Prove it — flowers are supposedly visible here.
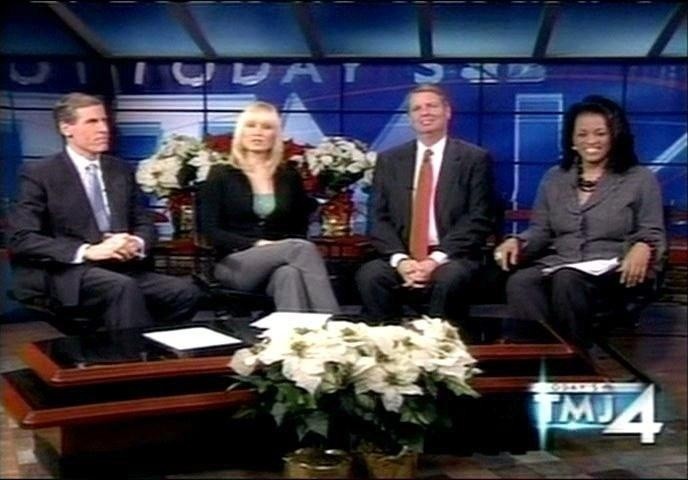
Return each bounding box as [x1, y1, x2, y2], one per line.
[289, 133, 380, 191]
[346, 318, 485, 453]
[130, 128, 238, 194]
[226, 317, 346, 440]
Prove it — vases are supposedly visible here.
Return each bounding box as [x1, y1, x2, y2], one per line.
[358, 436, 420, 479]
[281, 449, 355, 478]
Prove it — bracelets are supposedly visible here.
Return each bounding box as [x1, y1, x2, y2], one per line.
[631, 237, 657, 262]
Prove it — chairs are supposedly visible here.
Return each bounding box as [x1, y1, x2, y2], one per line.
[0, 180, 687, 346]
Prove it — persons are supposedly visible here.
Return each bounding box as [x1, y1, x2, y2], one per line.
[352, 83, 493, 323]
[494, 94, 667, 348]
[6, 93, 204, 330]
[197, 101, 340, 316]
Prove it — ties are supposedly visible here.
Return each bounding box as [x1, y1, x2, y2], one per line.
[409, 150, 433, 260]
[87, 165, 111, 233]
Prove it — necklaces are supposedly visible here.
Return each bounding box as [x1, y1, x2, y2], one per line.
[577, 166, 602, 192]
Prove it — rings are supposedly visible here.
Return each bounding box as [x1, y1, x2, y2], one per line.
[496, 252, 502, 260]
